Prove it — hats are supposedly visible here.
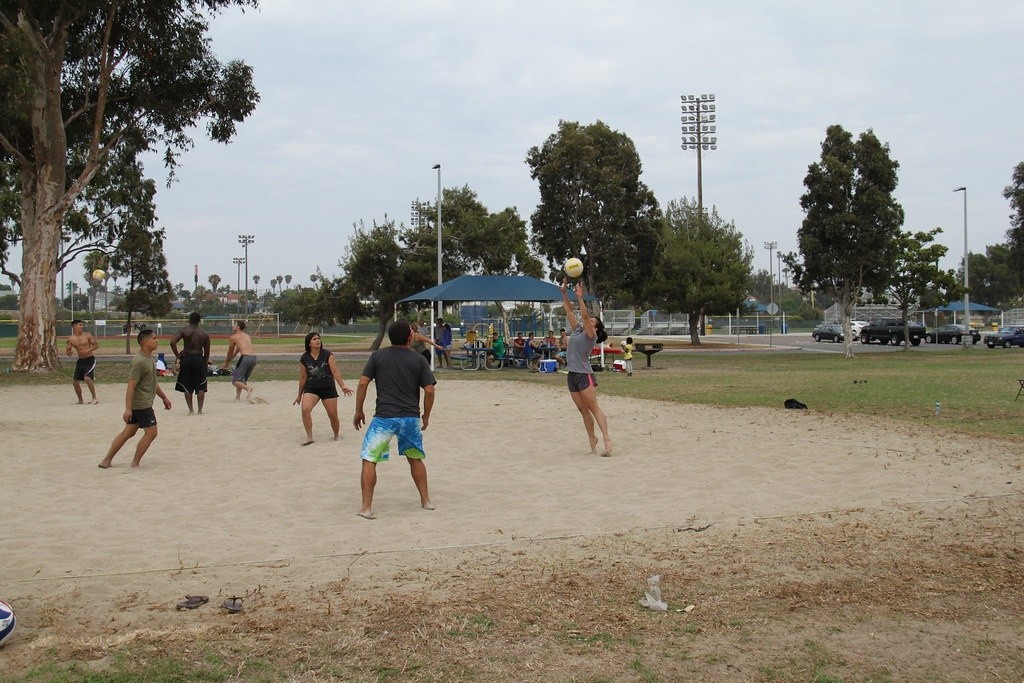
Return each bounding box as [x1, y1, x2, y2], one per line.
[436, 318, 443, 323]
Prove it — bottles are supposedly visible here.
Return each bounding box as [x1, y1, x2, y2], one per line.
[158, 353, 166, 368]
[474, 340, 477, 349]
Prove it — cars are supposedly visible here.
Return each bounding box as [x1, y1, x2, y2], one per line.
[983, 324, 1024, 349]
[924, 323, 982, 345]
[860, 317, 926, 347]
[840, 320, 871, 341]
[811, 323, 844, 342]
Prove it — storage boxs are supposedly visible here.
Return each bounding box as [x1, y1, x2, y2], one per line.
[540, 358, 557, 372]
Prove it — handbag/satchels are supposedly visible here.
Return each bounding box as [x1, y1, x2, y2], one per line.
[217, 369, 233, 377]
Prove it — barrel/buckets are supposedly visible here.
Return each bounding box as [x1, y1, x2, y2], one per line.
[467, 331, 476, 344]
[760, 324, 764, 333]
[706, 324, 712, 334]
[781, 324, 788, 334]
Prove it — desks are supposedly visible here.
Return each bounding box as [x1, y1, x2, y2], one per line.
[460, 346, 495, 371]
[590, 347, 622, 364]
[537, 347, 557, 361]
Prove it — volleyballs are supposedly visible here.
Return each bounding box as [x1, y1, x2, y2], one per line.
[564, 258, 583, 277]
[620, 341, 627, 346]
[93, 269, 106, 280]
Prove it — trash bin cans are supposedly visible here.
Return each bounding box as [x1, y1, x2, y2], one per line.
[781, 324, 788, 334]
[467, 330, 478, 344]
[760, 325, 764, 334]
[992, 322, 999, 331]
[706, 324, 713, 334]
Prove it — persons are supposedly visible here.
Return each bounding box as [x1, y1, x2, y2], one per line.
[353, 317, 436, 518]
[170, 312, 210, 415]
[98, 330, 171, 469]
[560, 278, 612, 457]
[66, 319, 99, 404]
[513, 333, 525, 355]
[543, 331, 556, 358]
[621, 337, 633, 376]
[123, 322, 141, 334]
[223, 320, 257, 400]
[552, 328, 569, 367]
[523, 332, 543, 368]
[471, 338, 485, 367]
[486, 332, 505, 368]
[410, 318, 452, 369]
[293, 332, 354, 446]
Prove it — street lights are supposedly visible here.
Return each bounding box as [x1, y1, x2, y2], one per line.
[432, 162, 444, 324]
[952, 186, 970, 334]
[680, 93, 718, 337]
[233, 257, 246, 319]
[763, 241, 778, 332]
[237, 235, 256, 324]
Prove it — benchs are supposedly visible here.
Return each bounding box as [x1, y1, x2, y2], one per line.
[451, 354, 535, 371]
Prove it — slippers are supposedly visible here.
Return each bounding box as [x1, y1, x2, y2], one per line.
[220, 595, 245, 614]
[98, 464, 107, 469]
[176, 594, 210, 612]
